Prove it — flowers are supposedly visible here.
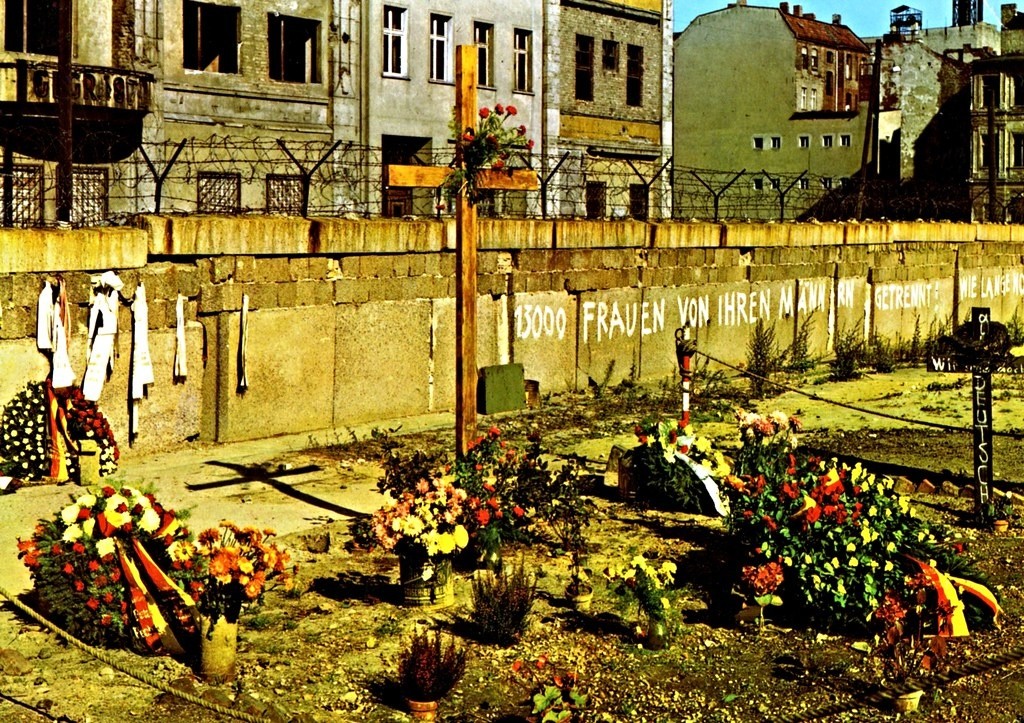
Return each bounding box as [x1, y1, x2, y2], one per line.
[565, 551, 593, 592]
[161, 521, 290, 639]
[355, 468, 468, 555]
[444, 103, 534, 194]
[375, 623, 470, 702]
[623, 560, 690, 613]
[876, 577, 955, 689]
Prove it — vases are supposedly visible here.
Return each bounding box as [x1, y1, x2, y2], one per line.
[410, 699, 437, 723]
[651, 618, 668, 648]
[892, 689, 923, 714]
[198, 617, 236, 682]
[400, 548, 454, 606]
[566, 586, 593, 613]
